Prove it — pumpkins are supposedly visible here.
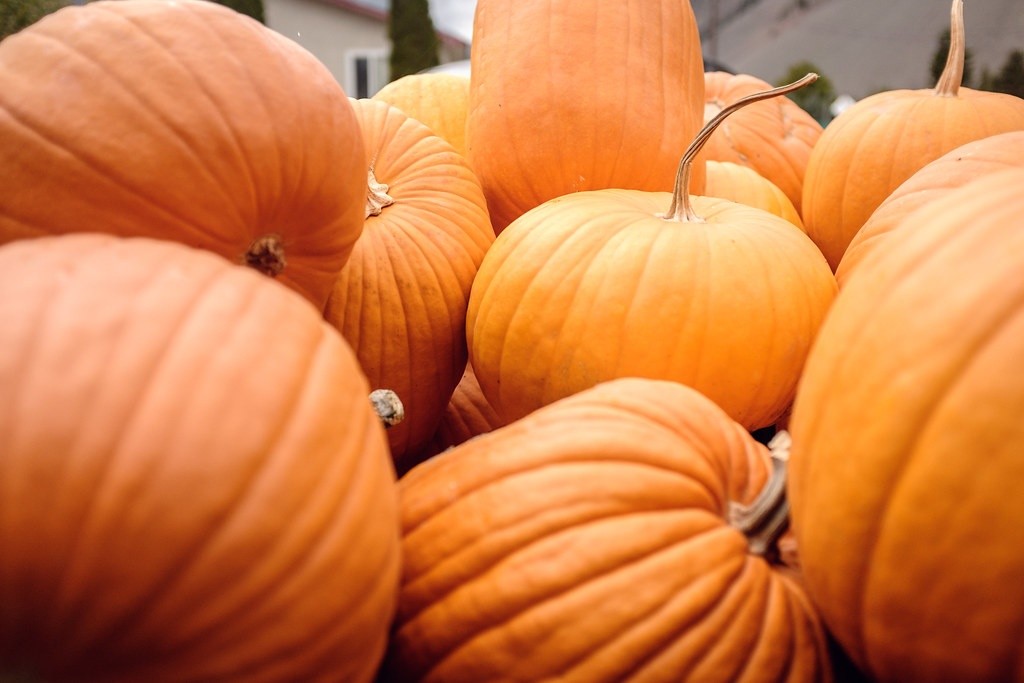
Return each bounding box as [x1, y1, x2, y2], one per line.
[0, 0, 1024, 683]
